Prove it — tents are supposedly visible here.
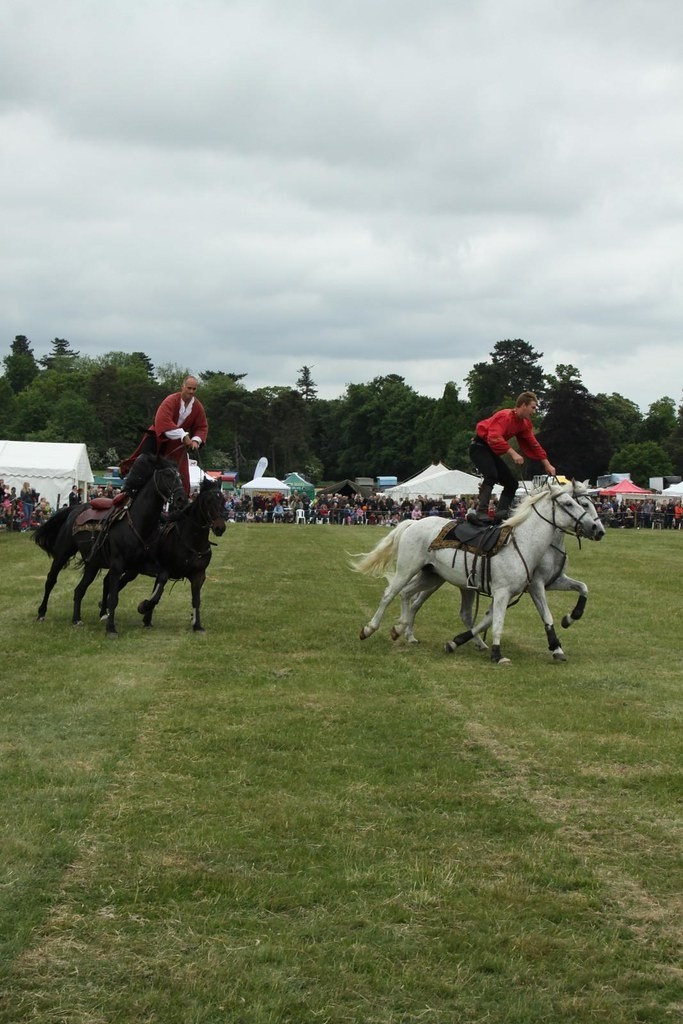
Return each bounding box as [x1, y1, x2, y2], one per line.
[0, 440, 95, 512]
[246, 462, 683, 508]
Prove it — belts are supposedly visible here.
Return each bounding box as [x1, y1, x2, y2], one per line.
[471, 438, 487, 446]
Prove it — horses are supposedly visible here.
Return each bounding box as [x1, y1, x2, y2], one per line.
[342, 478, 605, 668]
[29, 456, 228, 640]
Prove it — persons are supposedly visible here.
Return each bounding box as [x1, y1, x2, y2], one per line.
[153, 375, 209, 498]
[0, 478, 683, 532]
[468, 391, 558, 524]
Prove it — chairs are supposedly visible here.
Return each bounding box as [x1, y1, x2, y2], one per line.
[296, 509, 306, 524]
[650, 516, 662, 530]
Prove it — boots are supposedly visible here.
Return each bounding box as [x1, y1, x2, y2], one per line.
[476, 481, 493, 522]
[494, 495, 513, 524]
